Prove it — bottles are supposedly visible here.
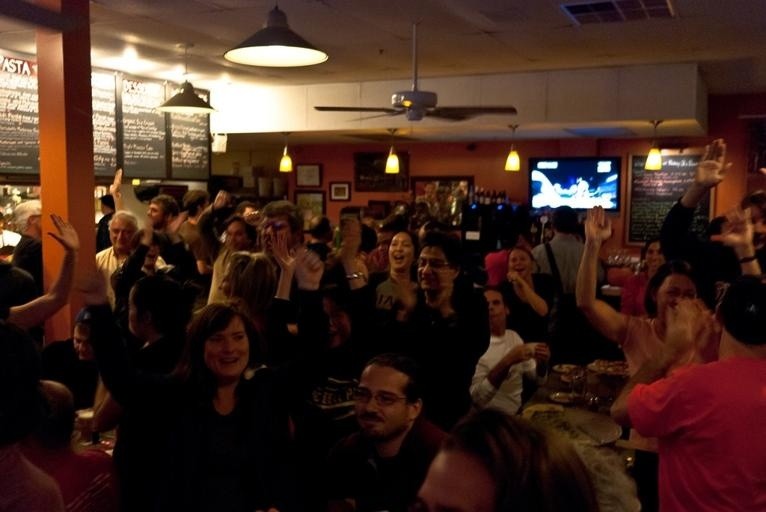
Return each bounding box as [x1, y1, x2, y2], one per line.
[467, 185, 506, 205]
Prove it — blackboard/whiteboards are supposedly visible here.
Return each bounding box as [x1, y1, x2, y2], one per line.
[625, 151, 716, 246]
[1, 49, 211, 184]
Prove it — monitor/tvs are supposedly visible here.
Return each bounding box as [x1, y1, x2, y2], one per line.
[528, 156, 622, 219]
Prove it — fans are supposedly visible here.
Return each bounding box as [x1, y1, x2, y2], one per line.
[311, 15, 517, 123]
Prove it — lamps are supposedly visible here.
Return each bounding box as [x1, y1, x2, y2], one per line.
[223, 0, 331, 67]
[154, 43, 218, 113]
[503, 125, 521, 172]
[277, 133, 293, 173]
[643, 120, 664, 171]
[383, 127, 400, 174]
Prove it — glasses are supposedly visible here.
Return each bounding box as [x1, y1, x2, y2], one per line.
[351, 386, 411, 408]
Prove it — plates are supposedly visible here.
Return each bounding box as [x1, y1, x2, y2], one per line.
[552, 363, 574, 372]
[587, 360, 627, 378]
[549, 391, 578, 403]
[520, 407, 623, 447]
[616, 438, 658, 453]
[258, 176, 287, 196]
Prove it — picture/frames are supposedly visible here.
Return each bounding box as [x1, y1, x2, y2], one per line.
[295, 163, 320, 186]
[412, 173, 474, 226]
[292, 190, 326, 221]
[328, 181, 351, 200]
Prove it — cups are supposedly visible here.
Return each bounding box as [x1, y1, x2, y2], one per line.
[570, 369, 587, 395]
[75, 407, 95, 447]
[527, 342, 540, 357]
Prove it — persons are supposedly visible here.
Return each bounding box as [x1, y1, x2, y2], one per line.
[657, 137, 766, 315]
[0, 166, 766, 512]
